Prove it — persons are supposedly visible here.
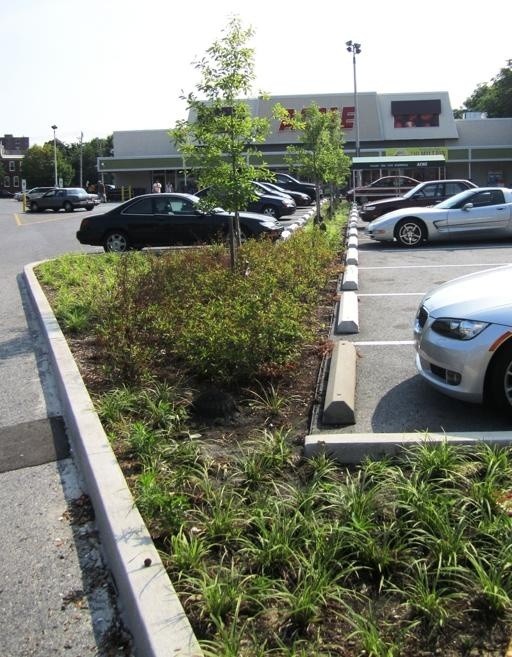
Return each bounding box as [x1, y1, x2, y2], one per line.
[180, 202, 193, 213]
[86, 180, 107, 203]
[152, 175, 173, 193]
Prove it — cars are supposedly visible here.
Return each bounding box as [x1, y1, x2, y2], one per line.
[0, 187, 14, 197]
[28, 186, 101, 212]
[191, 170, 325, 220]
[346, 174, 422, 204]
[360, 178, 483, 221]
[13, 188, 31, 201]
[413, 261, 512, 426]
[76, 191, 284, 252]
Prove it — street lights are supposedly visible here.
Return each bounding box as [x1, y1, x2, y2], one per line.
[51, 124, 60, 186]
[345, 38, 364, 188]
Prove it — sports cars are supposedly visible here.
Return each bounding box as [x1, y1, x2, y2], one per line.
[366, 186, 512, 247]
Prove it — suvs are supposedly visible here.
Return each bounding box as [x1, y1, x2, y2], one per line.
[19, 187, 60, 204]
